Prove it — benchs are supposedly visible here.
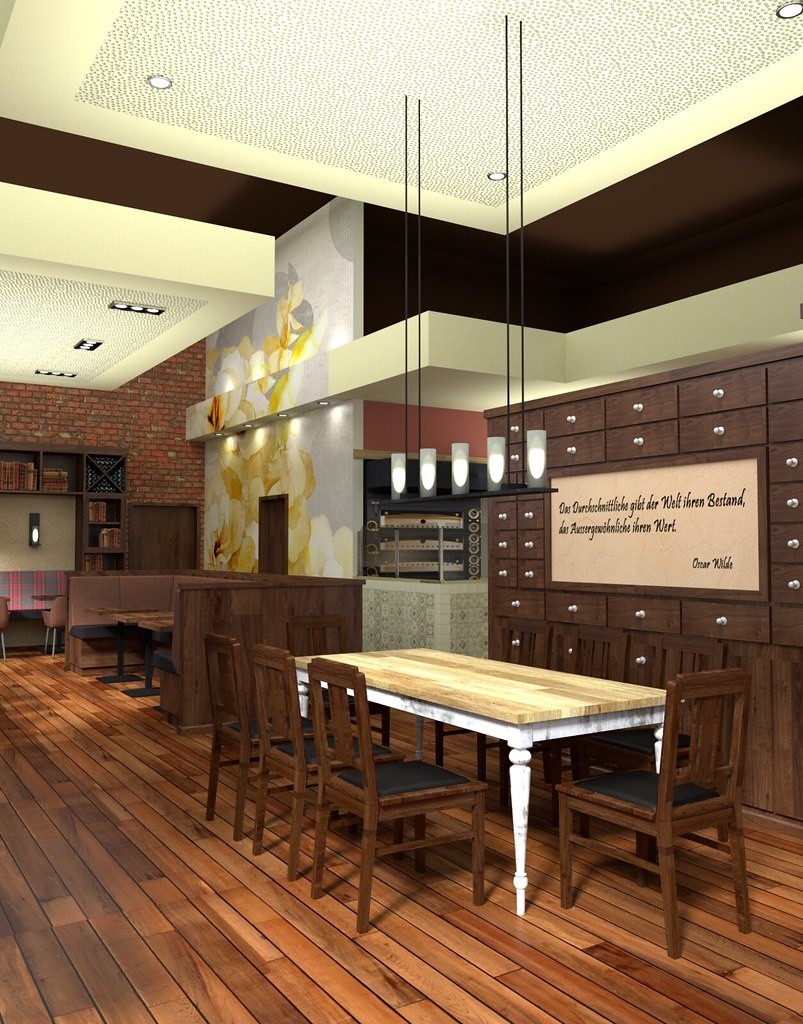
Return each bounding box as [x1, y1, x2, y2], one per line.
[64, 574, 366, 736]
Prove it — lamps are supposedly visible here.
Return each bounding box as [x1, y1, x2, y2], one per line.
[29, 513, 42, 547]
[377, 14, 559, 506]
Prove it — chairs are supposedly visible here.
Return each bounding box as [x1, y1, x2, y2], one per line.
[202, 614, 750, 960]
[42, 597, 68, 658]
[0, 598, 11, 662]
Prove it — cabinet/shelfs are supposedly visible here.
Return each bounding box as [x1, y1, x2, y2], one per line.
[0, 439, 129, 653]
[483, 343, 803, 822]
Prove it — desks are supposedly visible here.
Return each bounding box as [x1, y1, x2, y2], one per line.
[287, 646, 668, 920]
[111, 610, 174, 699]
[85, 607, 161, 685]
[138, 620, 174, 712]
[31, 595, 66, 654]
